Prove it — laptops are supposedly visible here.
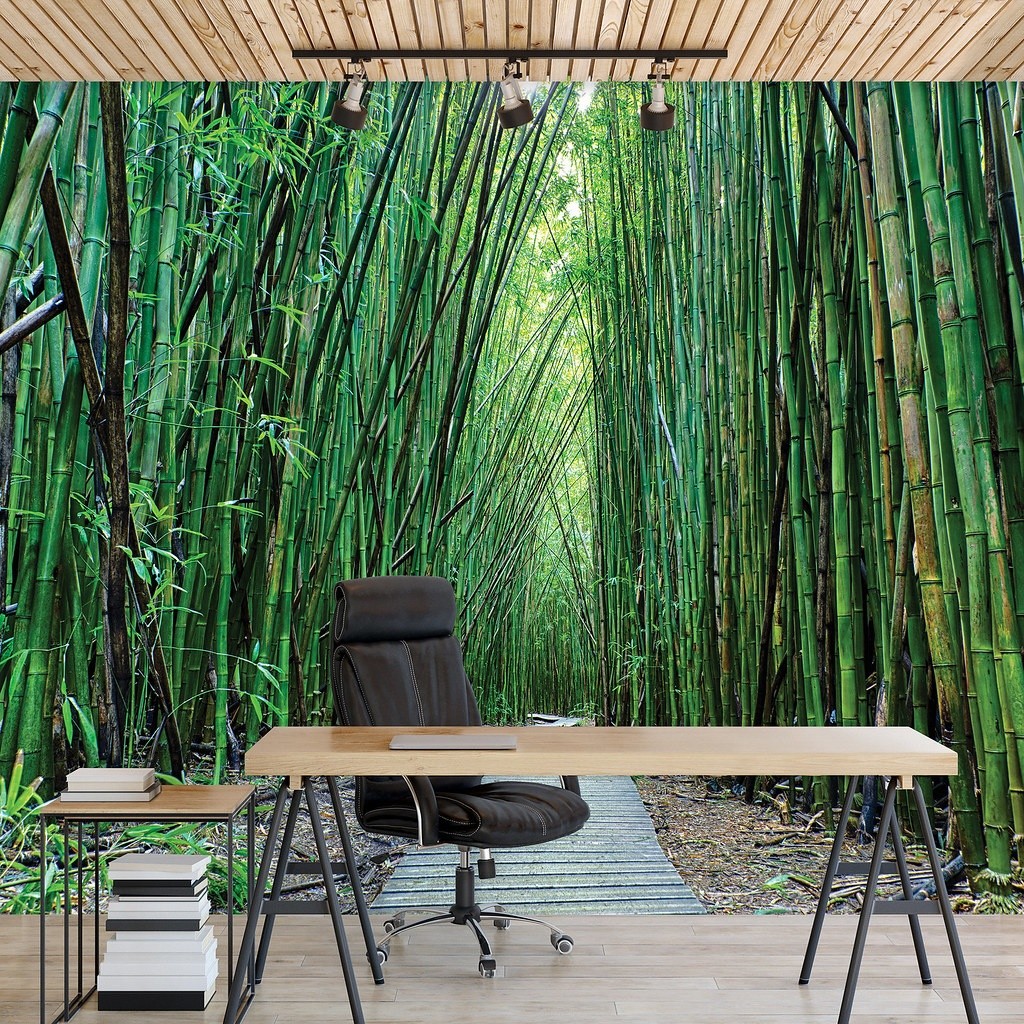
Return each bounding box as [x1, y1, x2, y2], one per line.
[389, 733, 516, 749]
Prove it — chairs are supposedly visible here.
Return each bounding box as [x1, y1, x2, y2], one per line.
[329, 575, 591, 978]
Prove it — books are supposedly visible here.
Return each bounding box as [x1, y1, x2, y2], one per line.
[97, 854, 219, 1011]
[60, 768, 162, 802]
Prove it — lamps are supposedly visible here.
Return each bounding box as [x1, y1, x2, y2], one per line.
[496, 57, 535, 130]
[332, 55, 367, 129]
[640, 57, 676, 131]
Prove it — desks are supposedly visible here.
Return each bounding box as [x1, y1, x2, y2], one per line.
[223, 724, 981, 1024]
[37, 785, 256, 1024]
